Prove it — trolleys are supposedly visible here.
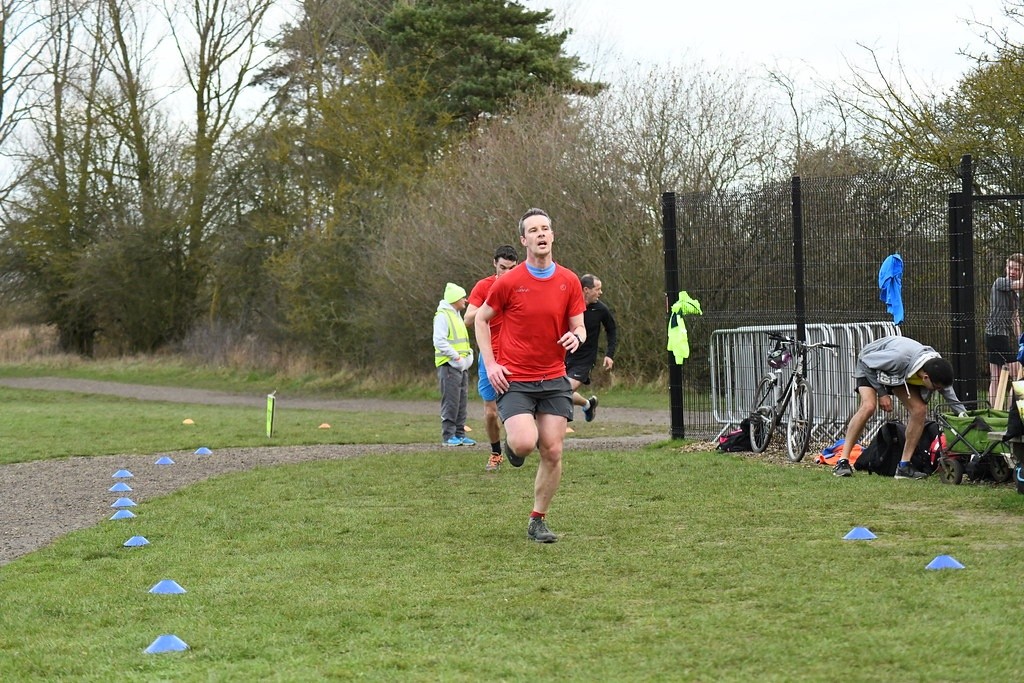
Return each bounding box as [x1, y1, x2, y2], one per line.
[935, 400, 1013, 483]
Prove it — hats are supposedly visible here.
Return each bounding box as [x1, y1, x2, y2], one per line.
[443, 283, 467, 304]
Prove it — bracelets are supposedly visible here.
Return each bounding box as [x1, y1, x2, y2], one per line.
[574, 334, 583, 346]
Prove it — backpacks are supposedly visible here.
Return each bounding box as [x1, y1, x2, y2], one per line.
[719, 417, 763, 453]
[852, 420, 947, 477]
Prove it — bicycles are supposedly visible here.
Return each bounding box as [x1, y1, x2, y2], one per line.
[749, 329, 841, 462]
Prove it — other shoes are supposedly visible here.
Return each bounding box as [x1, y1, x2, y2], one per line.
[565, 426, 577, 433]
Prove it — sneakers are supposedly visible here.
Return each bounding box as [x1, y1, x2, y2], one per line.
[582, 395, 598, 422]
[528, 515, 558, 542]
[442, 436, 464, 446]
[485, 451, 504, 472]
[457, 437, 476, 446]
[505, 439, 525, 467]
[893, 461, 928, 480]
[833, 457, 852, 477]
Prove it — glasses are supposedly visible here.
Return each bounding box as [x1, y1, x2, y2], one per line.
[928, 375, 944, 391]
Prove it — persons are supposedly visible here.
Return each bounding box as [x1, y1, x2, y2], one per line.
[432, 282, 477, 446]
[563, 273, 617, 422]
[831, 332, 968, 480]
[463, 245, 518, 472]
[984, 253, 1024, 411]
[474, 207, 588, 542]
